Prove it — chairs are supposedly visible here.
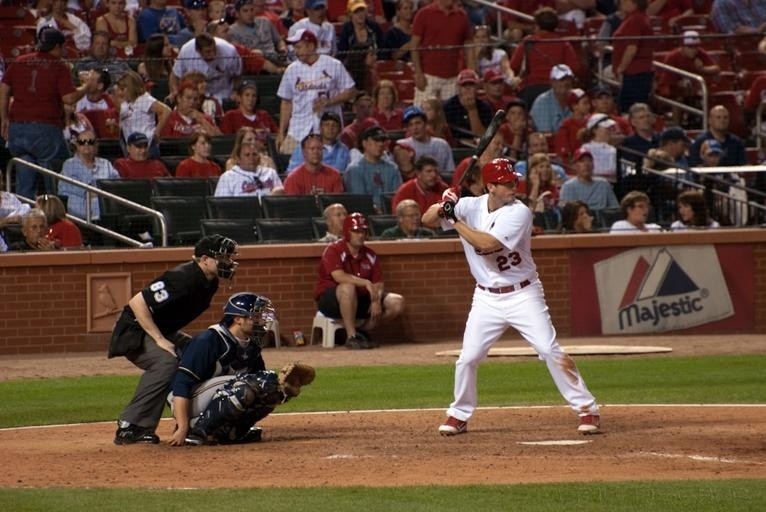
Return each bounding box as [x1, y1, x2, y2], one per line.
[1, 0, 766, 252]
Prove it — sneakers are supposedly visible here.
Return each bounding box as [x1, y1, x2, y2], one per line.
[113, 418, 160, 445]
[221, 426, 265, 445]
[346, 329, 380, 351]
[577, 413, 601, 434]
[437, 416, 468, 436]
[172, 425, 220, 446]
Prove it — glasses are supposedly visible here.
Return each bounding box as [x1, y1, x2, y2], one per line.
[74, 138, 99, 146]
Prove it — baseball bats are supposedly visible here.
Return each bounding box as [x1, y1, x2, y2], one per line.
[437, 109, 506, 220]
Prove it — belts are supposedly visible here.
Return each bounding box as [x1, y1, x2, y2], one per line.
[476, 279, 531, 296]
[7, 118, 59, 126]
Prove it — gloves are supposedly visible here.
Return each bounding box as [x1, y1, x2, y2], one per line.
[441, 186, 462, 226]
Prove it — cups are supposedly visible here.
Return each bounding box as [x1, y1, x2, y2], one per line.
[311, 99, 322, 114]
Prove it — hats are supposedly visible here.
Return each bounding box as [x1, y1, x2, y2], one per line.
[565, 84, 615, 108]
[125, 130, 149, 145]
[33, 26, 58, 53]
[457, 70, 481, 87]
[303, 0, 328, 11]
[569, 148, 594, 165]
[483, 67, 505, 83]
[175, 0, 263, 96]
[586, 112, 617, 133]
[355, 103, 428, 159]
[662, 126, 696, 145]
[321, 112, 342, 127]
[348, 0, 368, 13]
[681, 30, 702, 47]
[550, 63, 576, 81]
[699, 138, 727, 158]
[284, 28, 318, 46]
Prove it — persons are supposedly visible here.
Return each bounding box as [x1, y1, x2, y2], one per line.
[420, 156, 605, 435]
[316, 211, 403, 353]
[106, 234, 241, 446]
[165, 290, 318, 450]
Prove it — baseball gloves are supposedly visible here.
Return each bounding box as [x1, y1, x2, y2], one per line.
[275, 365, 316, 401]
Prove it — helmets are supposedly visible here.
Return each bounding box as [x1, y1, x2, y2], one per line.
[223, 292, 275, 345]
[481, 157, 523, 188]
[192, 233, 241, 280]
[342, 211, 371, 242]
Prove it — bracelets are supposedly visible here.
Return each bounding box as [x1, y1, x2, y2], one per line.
[448, 215, 461, 225]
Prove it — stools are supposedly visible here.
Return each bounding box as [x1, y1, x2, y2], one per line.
[308, 309, 366, 348]
[264, 319, 282, 348]
